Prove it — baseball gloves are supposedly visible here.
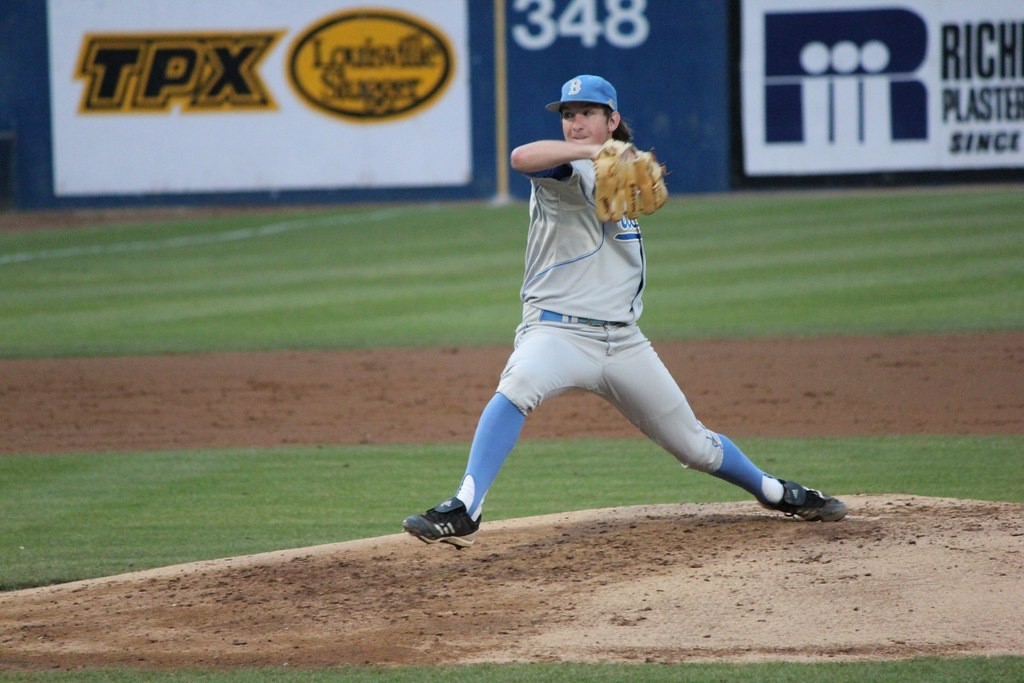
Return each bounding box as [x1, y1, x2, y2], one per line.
[592, 139, 669, 223]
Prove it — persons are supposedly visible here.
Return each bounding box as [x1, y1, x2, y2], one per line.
[403, 75, 848, 551]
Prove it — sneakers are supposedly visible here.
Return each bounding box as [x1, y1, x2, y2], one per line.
[402, 498, 482, 550]
[758, 477, 848, 521]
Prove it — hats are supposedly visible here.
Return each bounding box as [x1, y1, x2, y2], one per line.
[544, 74, 617, 113]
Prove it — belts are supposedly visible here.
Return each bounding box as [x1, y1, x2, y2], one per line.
[540, 310, 614, 327]
